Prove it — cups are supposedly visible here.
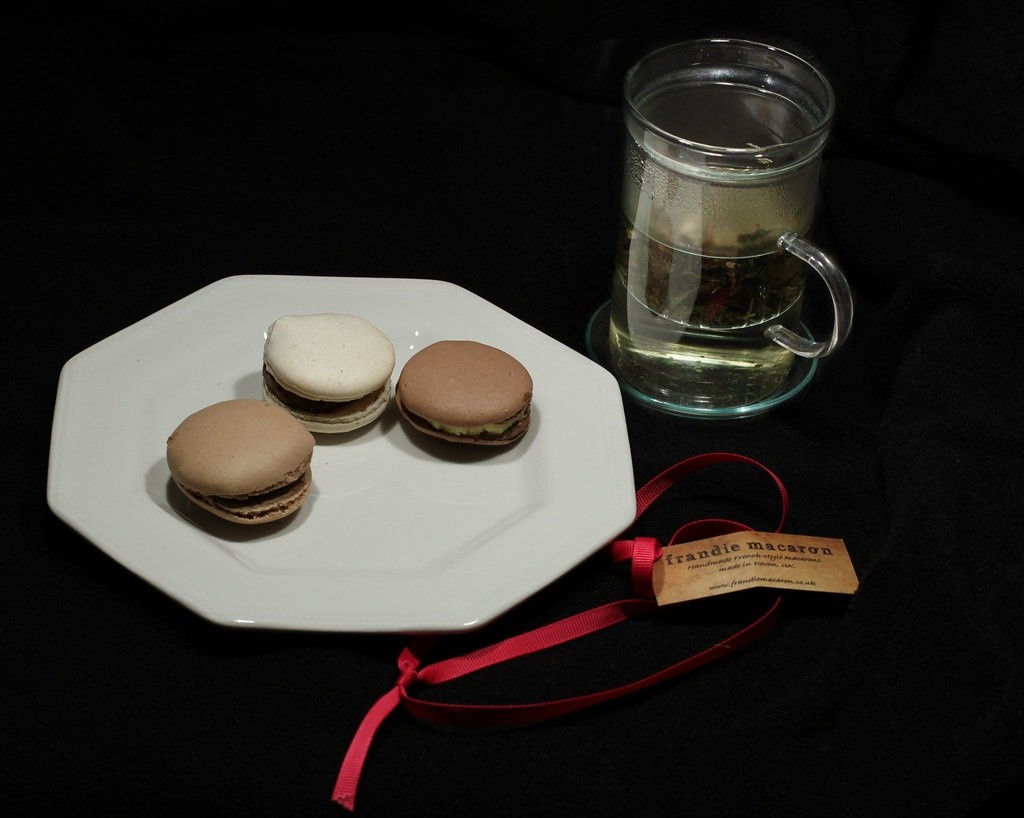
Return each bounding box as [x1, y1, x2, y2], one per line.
[605, 36, 854, 408]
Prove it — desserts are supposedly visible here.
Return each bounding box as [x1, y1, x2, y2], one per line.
[263, 314, 393, 434]
[166, 400, 316, 525]
[399, 340, 532, 458]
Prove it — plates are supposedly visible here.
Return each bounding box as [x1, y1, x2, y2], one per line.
[46, 275, 639, 632]
